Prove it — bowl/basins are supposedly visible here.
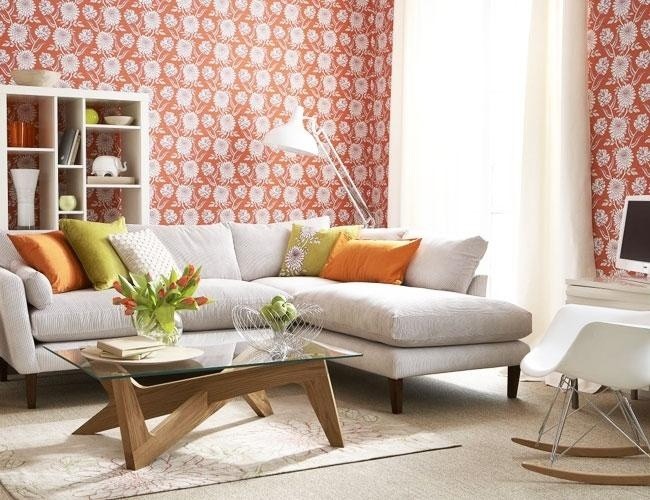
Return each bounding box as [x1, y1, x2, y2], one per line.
[12, 68, 61, 87]
[104, 116, 135, 126]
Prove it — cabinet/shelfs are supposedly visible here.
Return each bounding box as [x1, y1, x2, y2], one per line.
[0, 84, 150, 231]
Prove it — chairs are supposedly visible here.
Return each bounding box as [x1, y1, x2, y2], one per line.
[510, 301, 650, 487]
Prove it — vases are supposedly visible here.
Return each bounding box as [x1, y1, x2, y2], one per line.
[134, 310, 182, 345]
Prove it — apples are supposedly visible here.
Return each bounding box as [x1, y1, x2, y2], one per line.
[259, 297, 296, 333]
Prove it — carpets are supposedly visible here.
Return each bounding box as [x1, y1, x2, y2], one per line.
[1, 393, 463, 500]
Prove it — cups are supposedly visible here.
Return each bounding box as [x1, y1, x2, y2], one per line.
[59, 195, 78, 211]
[8, 119, 34, 147]
[7, 168, 41, 229]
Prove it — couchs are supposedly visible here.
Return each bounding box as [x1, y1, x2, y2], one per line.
[1, 225, 533, 414]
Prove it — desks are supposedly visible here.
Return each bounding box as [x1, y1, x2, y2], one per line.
[566, 279, 650, 409]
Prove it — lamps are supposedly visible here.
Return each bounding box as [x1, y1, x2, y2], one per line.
[263, 106, 376, 233]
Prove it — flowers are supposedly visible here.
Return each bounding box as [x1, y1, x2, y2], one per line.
[111, 264, 210, 321]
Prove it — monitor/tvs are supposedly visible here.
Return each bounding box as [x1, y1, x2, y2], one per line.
[615, 195, 650, 283]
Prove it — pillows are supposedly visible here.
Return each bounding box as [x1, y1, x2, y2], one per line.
[10, 217, 183, 294]
[278, 223, 423, 286]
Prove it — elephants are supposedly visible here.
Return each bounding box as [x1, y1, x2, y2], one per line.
[90, 155, 127, 177]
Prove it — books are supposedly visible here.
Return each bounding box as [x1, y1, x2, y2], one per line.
[97, 335, 165, 356]
[102, 350, 152, 361]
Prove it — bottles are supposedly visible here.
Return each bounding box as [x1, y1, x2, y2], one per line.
[86, 108, 98, 124]
